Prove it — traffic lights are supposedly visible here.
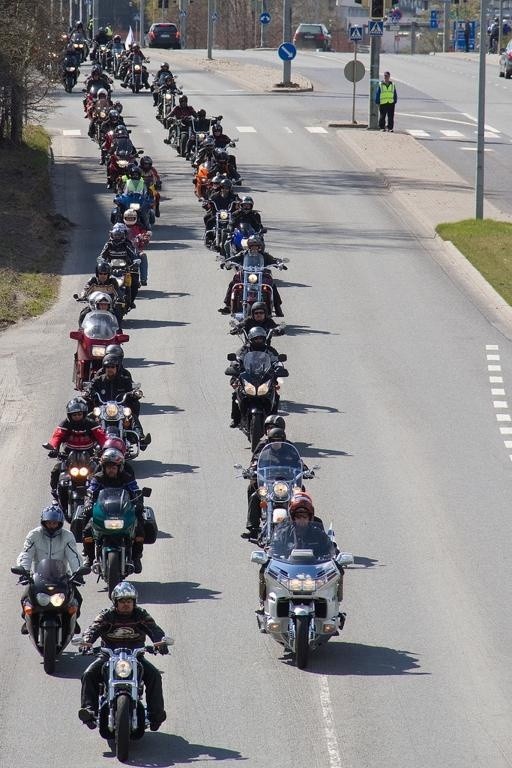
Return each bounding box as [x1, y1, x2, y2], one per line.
[158, 0, 162, 8]
[164, 0, 168, 8]
[385, 0, 400, 9]
[453, 0, 461, 6]
[462, 0, 468, 4]
[354, 0, 368, 7]
[370, 0, 384, 19]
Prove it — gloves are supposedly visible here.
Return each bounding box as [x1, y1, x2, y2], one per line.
[155, 644, 169, 655]
[48, 449, 58, 458]
[84, 499, 92, 514]
[303, 469, 314, 479]
[79, 645, 89, 656]
[135, 503, 143, 516]
[72, 572, 83, 587]
[19, 570, 30, 585]
[244, 466, 257, 480]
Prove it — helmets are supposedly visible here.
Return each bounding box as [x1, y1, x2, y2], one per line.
[91, 64, 129, 138]
[111, 582, 138, 606]
[41, 505, 64, 530]
[211, 177, 254, 215]
[287, 492, 313, 528]
[66, 397, 88, 422]
[101, 437, 127, 473]
[97, 263, 111, 280]
[248, 235, 264, 251]
[126, 156, 152, 179]
[264, 415, 286, 451]
[247, 326, 267, 347]
[89, 291, 113, 312]
[251, 302, 268, 322]
[160, 62, 187, 106]
[66, 21, 83, 54]
[102, 344, 124, 374]
[109, 208, 137, 243]
[196, 109, 222, 150]
[98, 27, 139, 53]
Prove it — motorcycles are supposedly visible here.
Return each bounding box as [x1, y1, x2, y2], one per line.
[225, 351, 287, 452]
[75, 484, 157, 598]
[70, 311, 132, 389]
[249, 522, 353, 668]
[233, 442, 321, 548]
[87, 390, 145, 464]
[43, 20, 150, 92]
[9, 558, 93, 675]
[42, 441, 109, 522]
[68, 634, 175, 761]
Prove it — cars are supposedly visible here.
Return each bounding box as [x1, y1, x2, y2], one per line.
[498, 39, 512, 78]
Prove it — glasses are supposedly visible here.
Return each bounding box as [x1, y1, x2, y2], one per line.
[119, 598, 132, 603]
[72, 413, 81, 416]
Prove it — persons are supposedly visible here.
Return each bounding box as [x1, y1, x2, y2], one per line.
[201, 174, 287, 357]
[229, 326, 343, 614]
[77, 155, 162, 332]
[77, 581, 168, 731]
[16, 345, 145, 636]
[28, 14, 149, 88]
[375, 71, 397, 132]
[456, 14, 512, 55]
[82, 61, 241, 187]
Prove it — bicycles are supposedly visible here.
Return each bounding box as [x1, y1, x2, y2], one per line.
[490, 37, 498, 54]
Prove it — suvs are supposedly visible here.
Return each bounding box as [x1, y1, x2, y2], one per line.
[146, 23, 181, 49]
[293, 23, 332, 51]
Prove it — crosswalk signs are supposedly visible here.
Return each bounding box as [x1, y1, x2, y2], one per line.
[350, 27, 363, 40]
[368, 20, 383, 36]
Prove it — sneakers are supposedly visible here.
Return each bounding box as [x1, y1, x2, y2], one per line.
[205, 233, 229, 258]
[79, 705, 97, 729]
[21, 622, 29, 634]
[150, 711, 165, 731]
[132, 553, 142, 573]
[74, 621, 81, 634]
[52, 497, 58, 505]
[338, 612, 346, 625]
[99, 153, 121, 194]
[230, 418, 240, 427]
[82, 549, 95, 567]
[140, 433, 150, 451]
[274, 305, 283, 316]
[217, 305, 231, 313]
[153, 101, 171, 129]
[163, 137, 195, 162]
[241, 527, 257, 539]
[255, 608, 265, 615]
